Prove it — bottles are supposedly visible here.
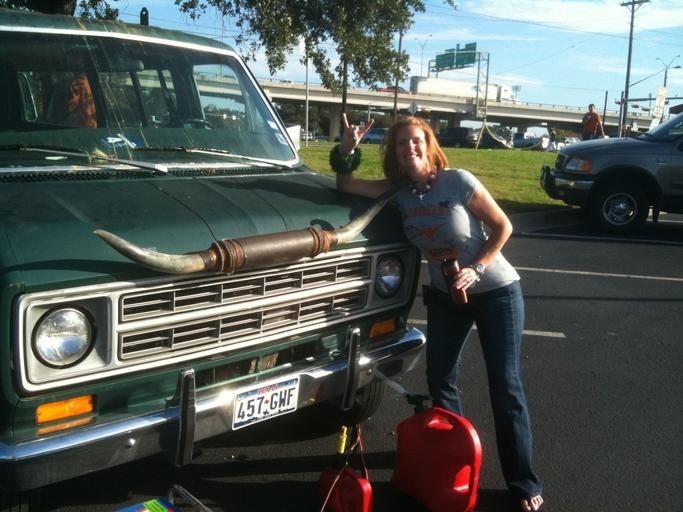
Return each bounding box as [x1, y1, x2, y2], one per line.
[441, 257, 470, 307]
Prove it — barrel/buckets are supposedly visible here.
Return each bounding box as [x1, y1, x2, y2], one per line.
[319, 463, 374, 512]
[391, 393, 482, 512]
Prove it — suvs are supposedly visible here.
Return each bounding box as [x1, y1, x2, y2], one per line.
[538, 112, 683, 237]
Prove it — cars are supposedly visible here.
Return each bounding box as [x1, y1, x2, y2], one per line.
[379, 83, 410, 95]
[280, 123, 578, 156]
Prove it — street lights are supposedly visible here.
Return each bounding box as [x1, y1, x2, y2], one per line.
[654, 55, 679, 89]
[411, 33, 432, 76]
[616, 66, 682, 137]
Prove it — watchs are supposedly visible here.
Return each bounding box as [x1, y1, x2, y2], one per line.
[463, 263, 486, 276]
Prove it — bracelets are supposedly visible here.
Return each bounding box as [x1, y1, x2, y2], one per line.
[329, 145, 362, 174]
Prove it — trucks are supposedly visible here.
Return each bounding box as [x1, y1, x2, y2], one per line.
[410, 76, 520, 104]
[0, 2, 425, 499]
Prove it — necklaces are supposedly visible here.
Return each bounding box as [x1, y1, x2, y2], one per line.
[402, 165, 438, 203]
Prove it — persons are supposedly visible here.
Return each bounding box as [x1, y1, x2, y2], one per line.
[335, 108, 545, 512]
[580, 103, 604, 141]
